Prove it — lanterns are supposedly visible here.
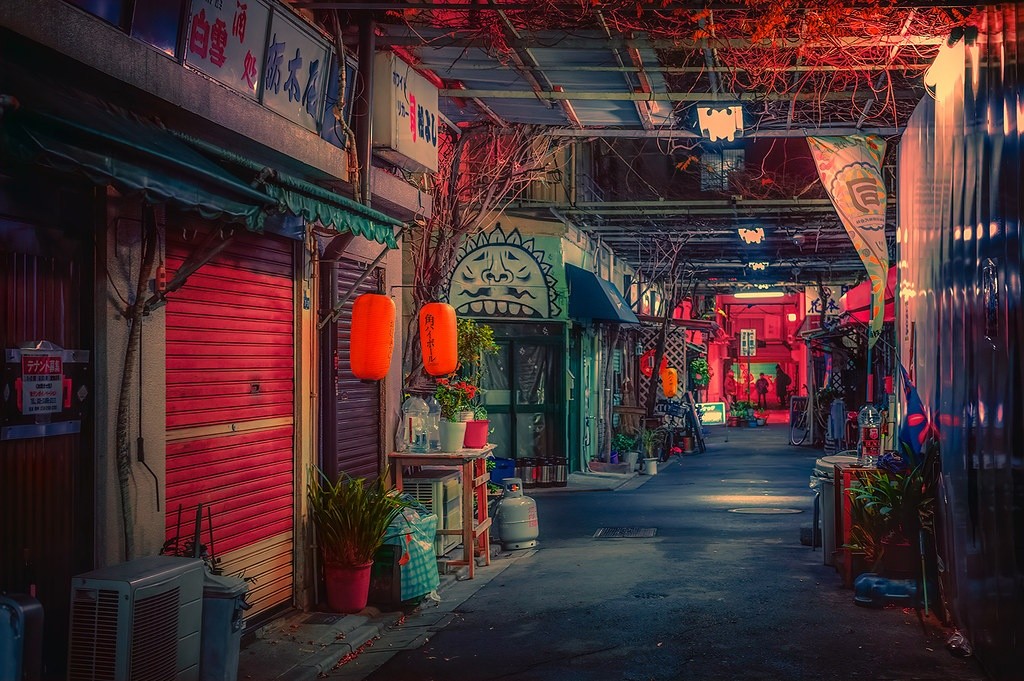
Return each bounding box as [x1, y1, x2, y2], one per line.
[419, 300, 458, 384]
[350, 290, 396, 385]
[662, 366, 677, 399]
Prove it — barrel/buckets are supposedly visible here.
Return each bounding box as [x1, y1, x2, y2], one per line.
[463, 420, 491, 448]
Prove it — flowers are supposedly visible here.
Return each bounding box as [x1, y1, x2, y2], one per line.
[432, 377, 478, 422]
[697, 278, 728, 323]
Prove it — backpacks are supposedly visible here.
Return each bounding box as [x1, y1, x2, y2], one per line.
[784, 373, 792, 386]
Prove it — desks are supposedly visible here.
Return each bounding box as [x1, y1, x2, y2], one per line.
[834, 462, 915, 590]
[380, 501, 439, 609]
[388, 442, 497, 578]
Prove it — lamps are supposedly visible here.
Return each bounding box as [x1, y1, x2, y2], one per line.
[738, 228, 766, 244]
[696, 103, 744, 143]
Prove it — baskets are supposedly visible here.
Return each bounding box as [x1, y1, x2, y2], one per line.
[795, 396, 808, 412]
[666, 403, 686, 419]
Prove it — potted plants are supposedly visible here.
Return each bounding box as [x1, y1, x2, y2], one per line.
[611, 434, 639, 473]
[434, 317, 501, 449]
[840, 441, 942, 577]
[303, 462, 425, 612]
[635, 426, 665, 475]
[728, 400, 766, 428]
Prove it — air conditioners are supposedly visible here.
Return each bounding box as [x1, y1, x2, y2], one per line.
[67, 553, 204, 681]
[402, 468, 464, 559]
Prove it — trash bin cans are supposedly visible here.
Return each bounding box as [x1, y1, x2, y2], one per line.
[202, 572, 254, 681]
[817, 456, 859, 568]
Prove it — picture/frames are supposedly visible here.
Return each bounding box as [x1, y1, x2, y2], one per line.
[697, 402, 726, 426]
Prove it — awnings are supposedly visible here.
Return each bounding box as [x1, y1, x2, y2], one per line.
[564, 261, 640, 324]
[0, 88, 409, 318]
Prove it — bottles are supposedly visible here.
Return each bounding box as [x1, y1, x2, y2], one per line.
[402, 392, 443, 455]
[856, 401, 882, 470]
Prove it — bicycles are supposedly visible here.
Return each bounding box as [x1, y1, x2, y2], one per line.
[777, 386, 798, 408]
[652, 396, 686, 462]
[791, 384, 827, 445]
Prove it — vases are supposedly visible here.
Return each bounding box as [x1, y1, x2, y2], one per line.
[438, 420, 466, 452]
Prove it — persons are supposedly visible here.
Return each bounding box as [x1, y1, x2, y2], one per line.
[755, 374, 769, 410]
[723, 370, 738, 402]
[775, 364, 786, 407]
